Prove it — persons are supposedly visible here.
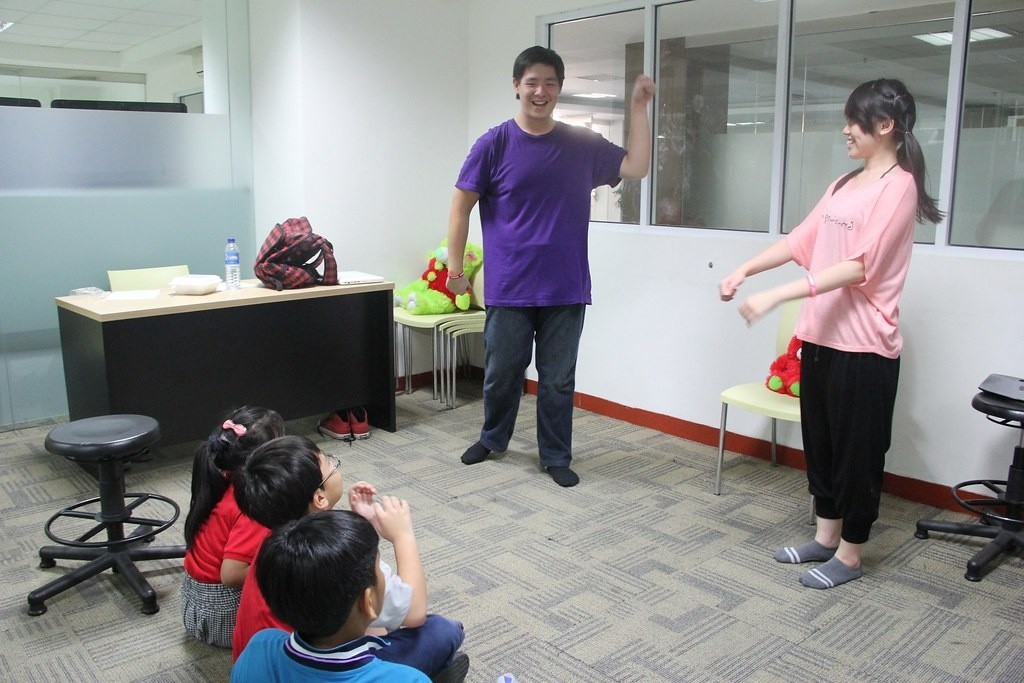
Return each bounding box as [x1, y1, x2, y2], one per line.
[444, 46, 657, 488]
[718, 78, 947, 591]
[182, 406, 470, 683]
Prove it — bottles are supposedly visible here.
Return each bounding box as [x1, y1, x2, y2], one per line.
[225, 238, 240, 291]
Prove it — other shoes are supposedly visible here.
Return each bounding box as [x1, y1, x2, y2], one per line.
[431, 651, 469, 683]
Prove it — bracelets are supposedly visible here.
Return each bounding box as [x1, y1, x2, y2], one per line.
[448, 270, 464, 280]
[806, 274, 817, 298]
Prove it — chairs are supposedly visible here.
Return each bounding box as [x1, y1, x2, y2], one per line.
[714, 297, 816, 525]
[107, 265, 190, 291]
[392, 262, 525, 410]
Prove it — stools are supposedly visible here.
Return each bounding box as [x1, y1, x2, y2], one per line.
[915, 392, 1024, 582]
[26, 415, 186, 618]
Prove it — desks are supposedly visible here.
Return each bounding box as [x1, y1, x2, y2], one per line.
[53, 271, 397, 483]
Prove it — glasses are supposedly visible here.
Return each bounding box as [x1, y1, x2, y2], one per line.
[319, 449, 341, 487]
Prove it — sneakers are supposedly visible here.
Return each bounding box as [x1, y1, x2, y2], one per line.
[316, 406, 352, 439]
[346, 407, 370, 439]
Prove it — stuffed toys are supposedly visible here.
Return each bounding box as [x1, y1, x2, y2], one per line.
[766, 336, 803, 398]
[392, 235, 484, 316]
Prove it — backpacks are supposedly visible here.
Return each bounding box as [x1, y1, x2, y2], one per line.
[254, 217, 337, 290]
